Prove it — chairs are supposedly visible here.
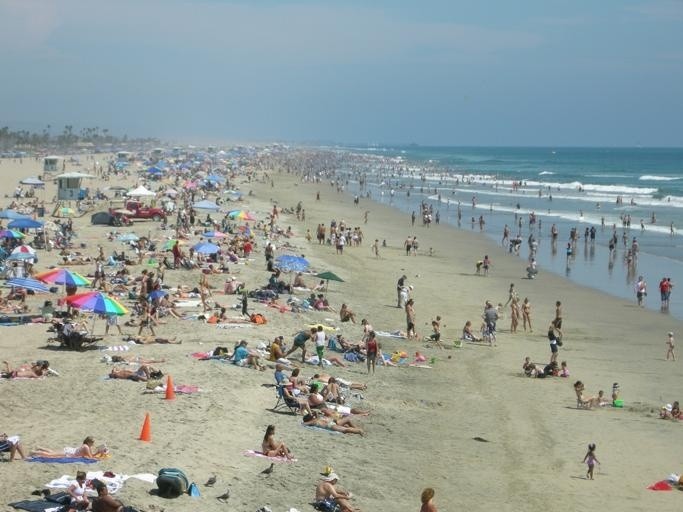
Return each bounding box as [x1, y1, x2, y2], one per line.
[47, 320, 102, 351]
[0, 301, 40, 325]
[271, 388, 301, 415]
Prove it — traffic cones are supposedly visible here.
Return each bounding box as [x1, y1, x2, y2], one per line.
[139, 413, 153, 442]
[165, 375, 177, 400]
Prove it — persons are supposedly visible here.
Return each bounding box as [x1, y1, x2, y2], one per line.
[1, 144, 683, 512]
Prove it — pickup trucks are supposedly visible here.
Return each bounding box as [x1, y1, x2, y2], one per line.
[109, 200, 165, 222]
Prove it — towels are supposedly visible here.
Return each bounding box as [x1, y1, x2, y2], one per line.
[27, 450, 111, 465]
[160, 279, 294, 331]
[297, 310, 410, 369]
[142, 382, 203, 395]
[243, 449, 298, 463]
[297, 420, 341, 438]
[8, 498, 68, 512]
[43, 471, 121, 496]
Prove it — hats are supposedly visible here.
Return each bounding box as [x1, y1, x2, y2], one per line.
[421, 488, 434, 502]
[279, 379, 292, 385]
[326, 473, 338, 481]
